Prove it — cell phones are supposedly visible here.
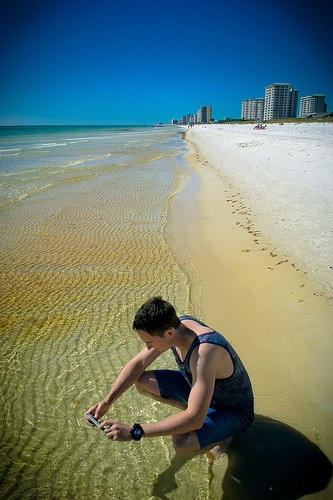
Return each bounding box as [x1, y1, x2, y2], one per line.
[87, 412, 106, 430]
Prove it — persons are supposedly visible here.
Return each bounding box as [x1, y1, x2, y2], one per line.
[84, 297, 256, 461]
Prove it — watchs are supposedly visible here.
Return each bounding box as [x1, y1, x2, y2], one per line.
[129, 422, 144, 442]
